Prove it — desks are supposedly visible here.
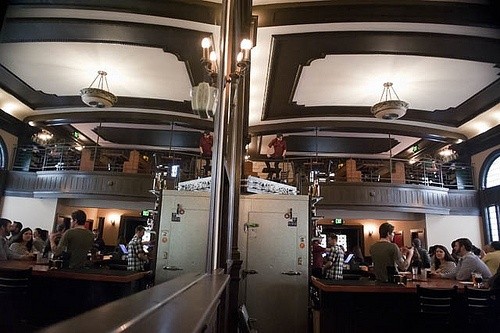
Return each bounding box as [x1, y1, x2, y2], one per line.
[0, 259, 152, 287]
[310, 279, 480, 333]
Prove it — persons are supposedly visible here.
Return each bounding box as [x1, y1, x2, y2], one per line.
[48, 210, 96, 272]
[370, 222, 414, 283]
[339, 245, 365, 270]
[114, 224, 148, 271]
[398, 240, 500, 282]
[0, 210, 104, 274]
[310, 237, 333, 298]
[324, 234, 344, 279]
[199, 132, 213, 174]
[265, 133, 287, 180]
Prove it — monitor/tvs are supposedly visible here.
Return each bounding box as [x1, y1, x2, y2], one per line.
[120, 244, 128, 254]
[344, 254, 354, 263]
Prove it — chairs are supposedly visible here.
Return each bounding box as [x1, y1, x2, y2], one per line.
[463, 285, 493, 333]
[243, 160, 257, 179]
[416, 285, 457, 333]
[316, 159, 332, 183]
[0, 267, 32, 328]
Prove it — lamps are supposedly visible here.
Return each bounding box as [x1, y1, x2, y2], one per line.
[200, 35, 218, 81]
[229, 38, 254, 83]
[370, 82, 409, 120]
[80, 71, 119, 109]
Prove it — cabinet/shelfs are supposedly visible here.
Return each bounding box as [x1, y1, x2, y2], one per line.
[123, 151, 155, 174]
[79, 148, 107, 171]
[336, 159, 362, 182]
[379, 161, 405, 184]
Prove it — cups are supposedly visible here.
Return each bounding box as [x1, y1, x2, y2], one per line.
[471, 273, 476, 286]
[475, 272, 482, 285]
[421, 268, 427, 279]
[412, 266, 418, 275]
